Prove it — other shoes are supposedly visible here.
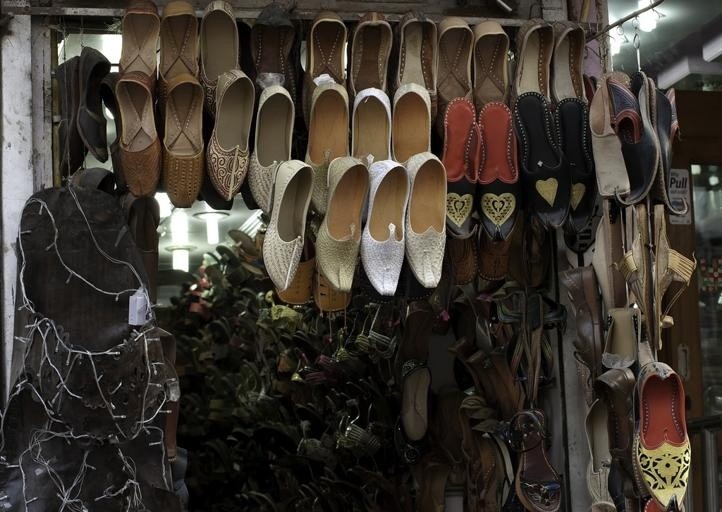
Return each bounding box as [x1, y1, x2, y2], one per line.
[314, 155, 370, 294]
[251, 3, 299, 112]
[473, 20, 511, 120]
[301, 7, 346, 127]
[351, 88, 392, 168]
[549, 20, 589, 109]
[164, 2, 200, 82]
[205, 69, 256, 204]
[249, 85, 296, 216]
[440, 18, 474, 117]
[403, 152, 448, 289]
[171, 231, 634, 510]
[262, 160, 315, 293]
[119, 5, 161, 101]
[200, 1, 243, 120]
[398, 11, 439, 119]
[350, 11, 396, 97]
[637, 360, 691, 509]
[360, 159, 411, 297]
[115, 72, 162, 200]
[392, 82, 433, 164]
[305, 82, 351, 216]
[161, 74, 205, 208]
[588, 69, 699, 351]
[511, 18, 555, 109]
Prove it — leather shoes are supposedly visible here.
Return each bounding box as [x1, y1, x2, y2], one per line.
[55, 56, 88, 177]
[71, 164, 117, 198]
[75, 45, 115, 164]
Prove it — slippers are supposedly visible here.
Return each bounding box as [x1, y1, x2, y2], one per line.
[479, 102, 519, 246]
[555, 97, 598, 231]
[440, 97, 478, 239]
[514, 91, 573, 232]
